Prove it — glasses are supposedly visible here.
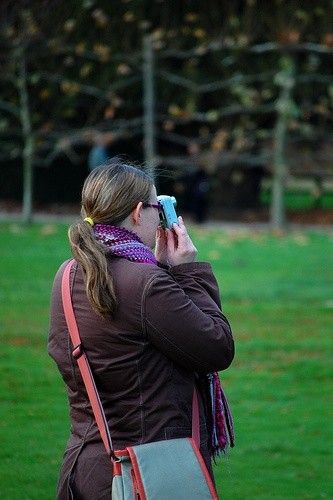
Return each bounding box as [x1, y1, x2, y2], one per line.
[143, 202, 163, 213]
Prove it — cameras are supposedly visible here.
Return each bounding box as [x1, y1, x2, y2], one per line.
[157, 195, 179, 231]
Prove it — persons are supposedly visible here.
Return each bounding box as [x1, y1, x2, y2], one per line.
[48, 163, 236, 499]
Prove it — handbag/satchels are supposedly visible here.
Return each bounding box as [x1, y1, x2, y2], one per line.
[111, 437, 218, 500]
